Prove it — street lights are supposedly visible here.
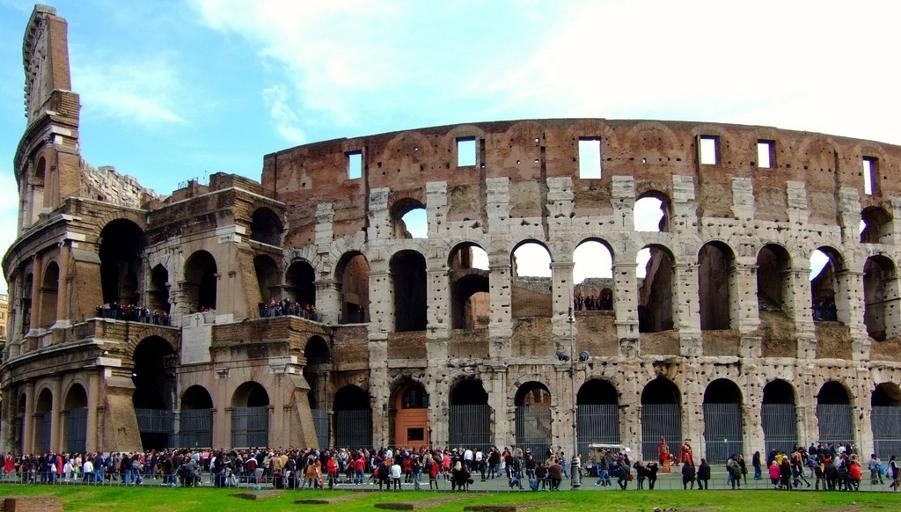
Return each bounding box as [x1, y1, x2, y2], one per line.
[326, 410, 335, 450]
[555, 304, 590, 489]
[425, 419, 432, 450]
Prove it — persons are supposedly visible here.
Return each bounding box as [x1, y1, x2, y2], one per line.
[0, 440, 900, 491]
[263, 296, 319, 323]
[94, 302, 169, 327]
